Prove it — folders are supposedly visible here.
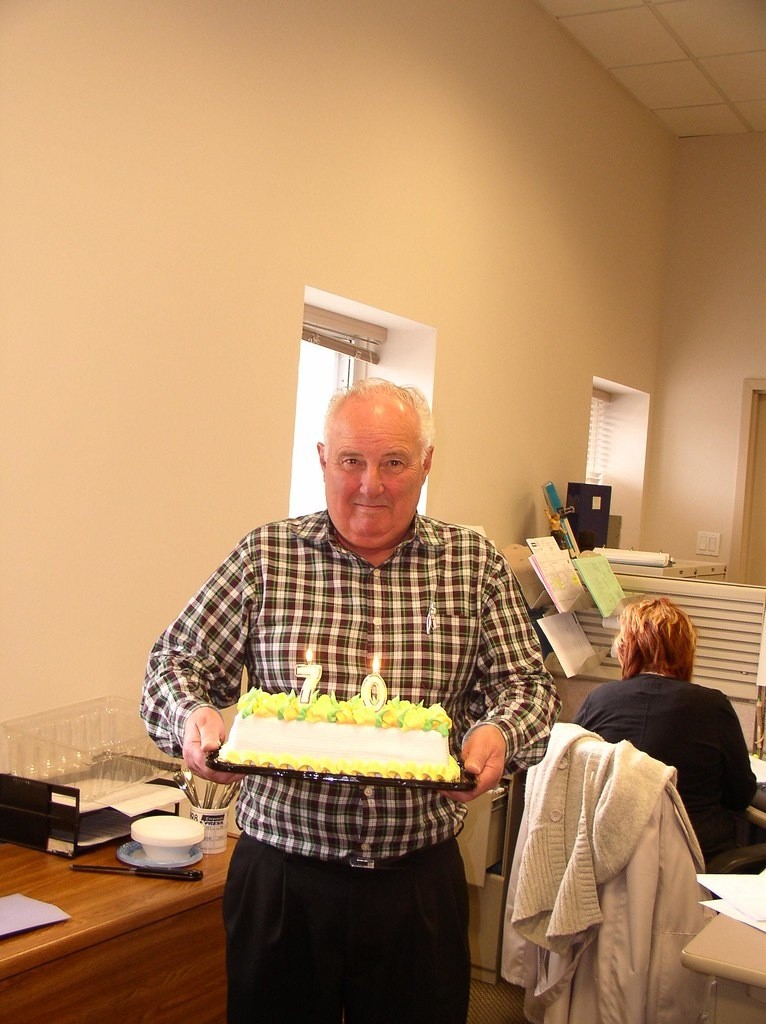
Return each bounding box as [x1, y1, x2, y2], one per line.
[565, 481, 612, 552]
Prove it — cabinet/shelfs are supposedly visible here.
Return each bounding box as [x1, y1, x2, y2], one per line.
[457, 770, 527, 983]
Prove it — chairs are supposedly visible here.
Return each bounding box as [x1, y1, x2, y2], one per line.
[500, 722, 766, 1024]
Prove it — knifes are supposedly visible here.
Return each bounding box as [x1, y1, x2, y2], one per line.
[70, 863, 204, 881]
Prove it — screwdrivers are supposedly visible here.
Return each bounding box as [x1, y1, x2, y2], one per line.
[66, 863, 204, 881]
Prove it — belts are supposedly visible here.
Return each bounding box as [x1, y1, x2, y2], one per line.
[297, 850, 428, 883]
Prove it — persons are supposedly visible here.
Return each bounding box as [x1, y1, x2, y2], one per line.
[139, 378, 561, 1024]
[544, 506, 566, 547]
[572, 595, 757, 860]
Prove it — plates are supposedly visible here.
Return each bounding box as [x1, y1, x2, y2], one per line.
[118, 841, 204, 869]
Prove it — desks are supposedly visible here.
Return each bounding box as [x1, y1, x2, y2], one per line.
[0, 832, 240, 1024]
[605, 558, 727, 581]
[681, 869, 766, 1024]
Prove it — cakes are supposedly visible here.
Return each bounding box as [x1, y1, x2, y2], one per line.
[217, 687, 460, 781]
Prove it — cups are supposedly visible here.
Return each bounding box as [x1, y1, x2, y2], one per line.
[189, 800, 230, 854]
